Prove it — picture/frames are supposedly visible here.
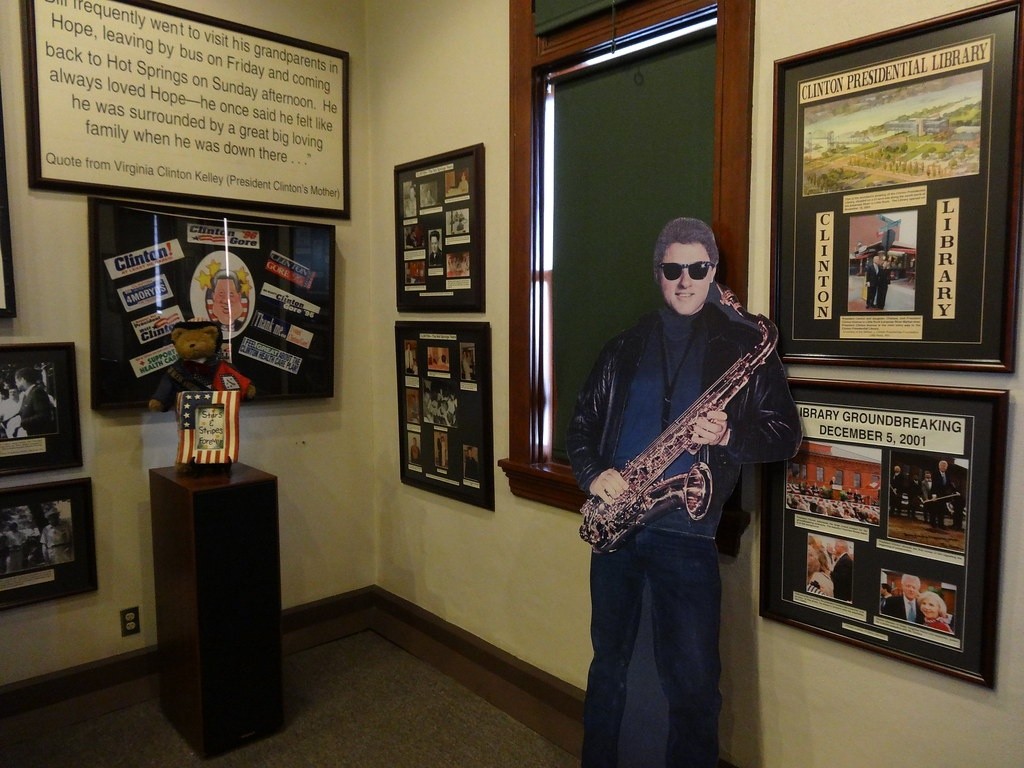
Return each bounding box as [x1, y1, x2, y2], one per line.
[769, 0, 1024, 371]
[758, 376, 1012, 691]
[394, 321, 496, 513]
[393, 142, 486, 314]
[0, 0, 350, 616]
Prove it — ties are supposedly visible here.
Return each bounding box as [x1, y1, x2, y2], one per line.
[907, 601, 916, 623]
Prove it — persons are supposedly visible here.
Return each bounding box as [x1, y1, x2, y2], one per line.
[0, 367, 55, 437]
[424, 388, 457, 426]
[429, 231, 442, 265]
[787, 477, 881, 525]
[866, 254, 891, 308]
[40, 508, 71, 563]
[410, 437, 421, 465]
[881, 574, 954, 634]
[406, 344, 414, 372]
[890, 460, 964, 530]
[565, 219, 802, 768]
[437, 433, 446, 467]
[806, 536, 852, 602]
[6, 522, 25, 573]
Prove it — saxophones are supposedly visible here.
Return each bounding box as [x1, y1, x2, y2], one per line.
[576, 279, 780, 554]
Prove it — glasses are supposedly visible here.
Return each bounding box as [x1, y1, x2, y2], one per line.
[658, 261, 715, 280]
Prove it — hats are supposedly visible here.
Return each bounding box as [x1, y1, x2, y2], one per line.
[44, 506, 61, 519]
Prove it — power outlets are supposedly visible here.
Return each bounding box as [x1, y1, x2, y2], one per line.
[118, 606, 141, 638]
